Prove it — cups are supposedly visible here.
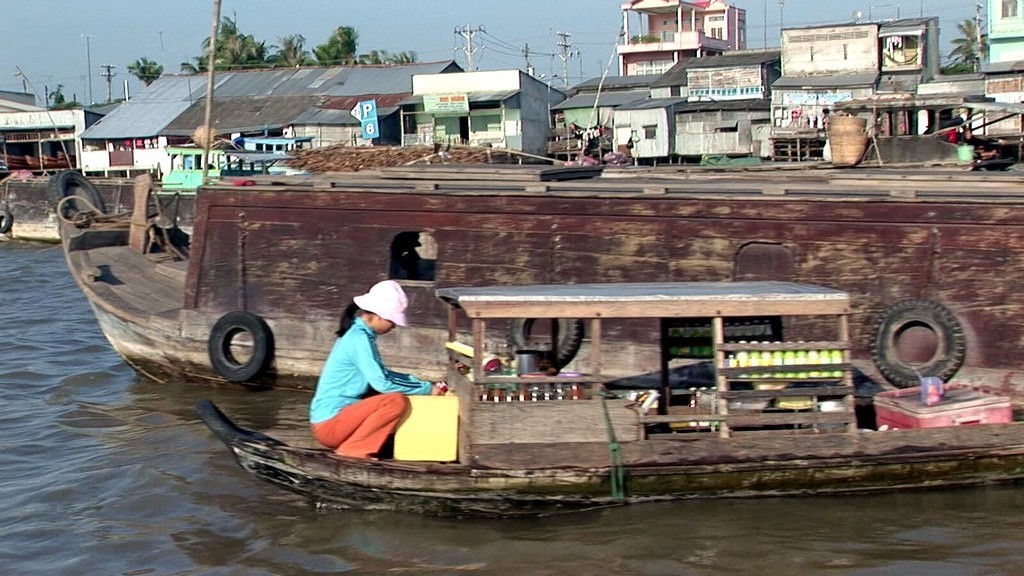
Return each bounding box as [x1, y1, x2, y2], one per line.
[920, 378, 944, 406]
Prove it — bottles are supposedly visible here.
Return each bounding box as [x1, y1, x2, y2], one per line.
[667, 320, 843, 381]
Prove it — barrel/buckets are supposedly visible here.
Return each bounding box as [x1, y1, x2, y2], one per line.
[956, 143, 976, 160]
[517, 351, 549, 374]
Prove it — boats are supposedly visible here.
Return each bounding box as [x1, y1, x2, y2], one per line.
[1, 0, 1024, 409]
[188, 273, 1024, 520]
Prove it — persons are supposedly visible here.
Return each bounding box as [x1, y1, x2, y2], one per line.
[309, 279, 448, 464]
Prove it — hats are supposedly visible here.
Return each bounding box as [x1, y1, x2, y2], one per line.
[353, 280, 409, 328]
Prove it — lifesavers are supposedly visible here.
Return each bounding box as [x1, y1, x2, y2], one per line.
[0, 211, 12, 233]
[868, 299, 967, 387]
[48, 171, 107, 217]
[505, 316, 583, 369]
[208, 311, 274, 382]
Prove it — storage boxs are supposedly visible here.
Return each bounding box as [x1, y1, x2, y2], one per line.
[394, 386, 459, 461]
[872, 388, 1013, 429]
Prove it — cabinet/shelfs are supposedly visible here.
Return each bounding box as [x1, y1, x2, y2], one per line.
[660, 317, 855, 433]
